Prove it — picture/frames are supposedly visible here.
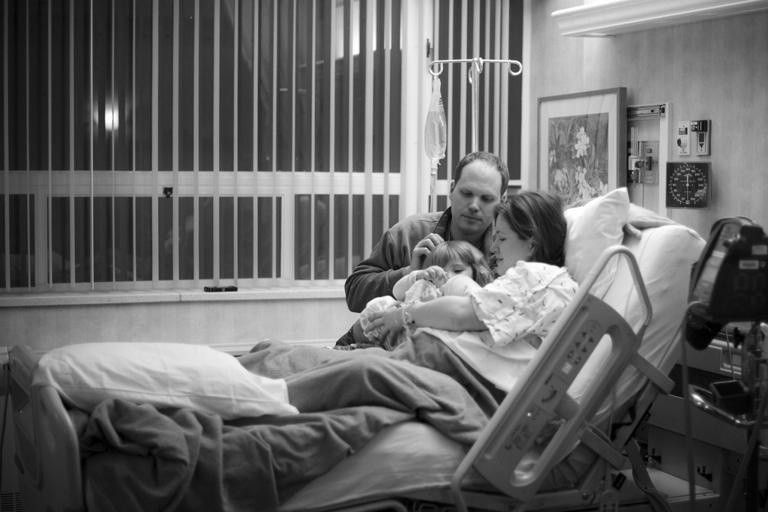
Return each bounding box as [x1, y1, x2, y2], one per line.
[536, 87, 628, 206]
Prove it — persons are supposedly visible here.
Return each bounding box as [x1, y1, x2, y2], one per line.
[365, 188, 580, 411]
[357, 273, 483, 352]
[344, 151, 510, 314]
[333, 238, 493, 353]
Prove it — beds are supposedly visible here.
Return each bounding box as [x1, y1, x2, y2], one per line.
[3, 188, 702, 511]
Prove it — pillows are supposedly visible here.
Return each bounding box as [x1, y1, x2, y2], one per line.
[563, 188, 629, 300]
